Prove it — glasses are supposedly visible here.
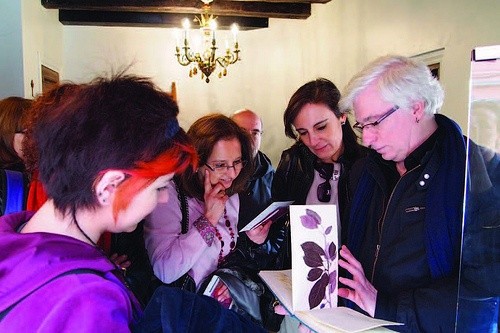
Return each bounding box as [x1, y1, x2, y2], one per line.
[199, 159, 247, 175]
[353, 104, 400, 134]
[316, 162, 335, 203]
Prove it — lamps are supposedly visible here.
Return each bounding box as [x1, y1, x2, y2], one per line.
[173, 0, 242, 85]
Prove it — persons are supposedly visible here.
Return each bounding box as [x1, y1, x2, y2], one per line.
[244, 77, 400, 333]
[458, 98, 500, 333]
[224, 107, 277, 270]
[0, 71, 190, 333]
[0, 95, 44, 226]
[137, 112, 254, 332]
[273, 52, 500, 333]
[19, 81, 85, 180]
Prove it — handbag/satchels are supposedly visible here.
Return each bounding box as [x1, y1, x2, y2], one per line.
[127, 175, 198, 310]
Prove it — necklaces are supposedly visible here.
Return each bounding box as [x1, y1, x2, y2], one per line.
[214, 204, 236, 265]
